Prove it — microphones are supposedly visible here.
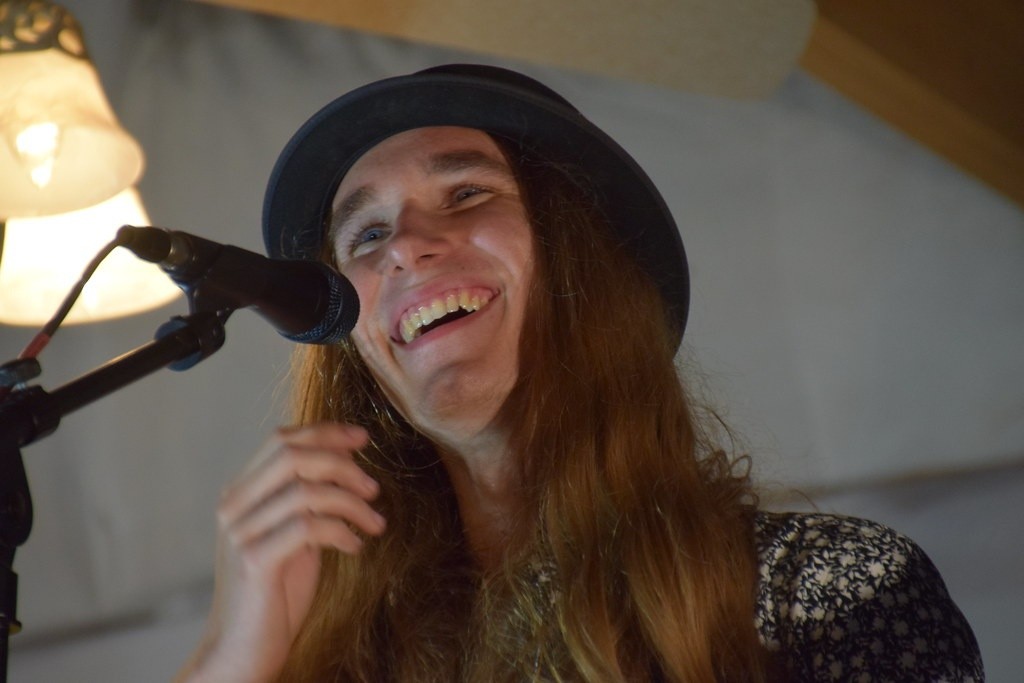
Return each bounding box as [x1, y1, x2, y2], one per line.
[117, 226, 359, 344]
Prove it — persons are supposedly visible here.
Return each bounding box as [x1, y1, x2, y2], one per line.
[157, 63, 983, 683]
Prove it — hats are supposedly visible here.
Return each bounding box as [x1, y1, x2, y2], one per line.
[262, 64, 691, 355]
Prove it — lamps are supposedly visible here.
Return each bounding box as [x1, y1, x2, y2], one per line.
[0, 0, 144, 222]
[0, 186, 188, 332]
